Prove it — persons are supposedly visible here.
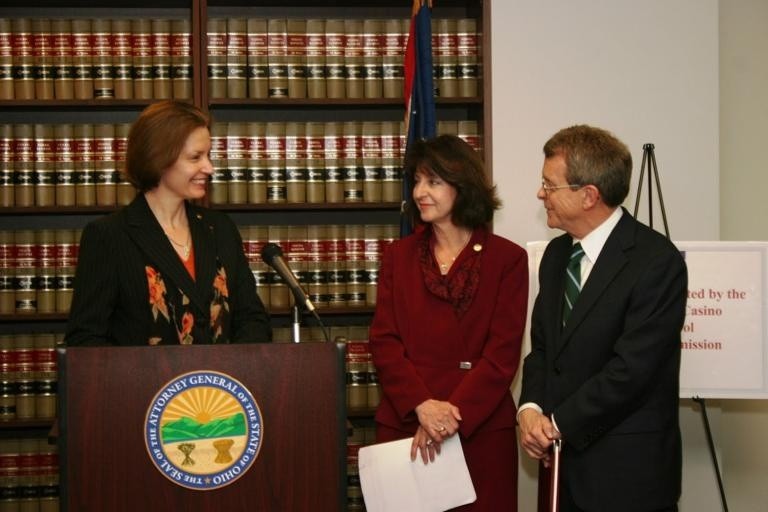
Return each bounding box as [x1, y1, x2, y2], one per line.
[360, 134, 527, 511]
[514, 124, 691, 511]
[64, 98, 274, 344]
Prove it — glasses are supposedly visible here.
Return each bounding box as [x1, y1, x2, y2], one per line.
[541, 176, 580, 197]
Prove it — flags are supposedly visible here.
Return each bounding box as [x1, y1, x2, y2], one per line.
[400, 1, 441, 240]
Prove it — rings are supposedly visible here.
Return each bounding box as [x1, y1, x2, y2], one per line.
[426, 438, 433, 445]
[437, 425, 445, 434]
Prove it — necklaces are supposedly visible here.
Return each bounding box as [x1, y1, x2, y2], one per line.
[436, 231, 468, 270]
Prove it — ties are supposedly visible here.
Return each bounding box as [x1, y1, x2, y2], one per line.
[560, 242, 584, 331]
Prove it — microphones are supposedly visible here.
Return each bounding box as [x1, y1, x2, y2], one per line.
[260, 242, 316, 314]
